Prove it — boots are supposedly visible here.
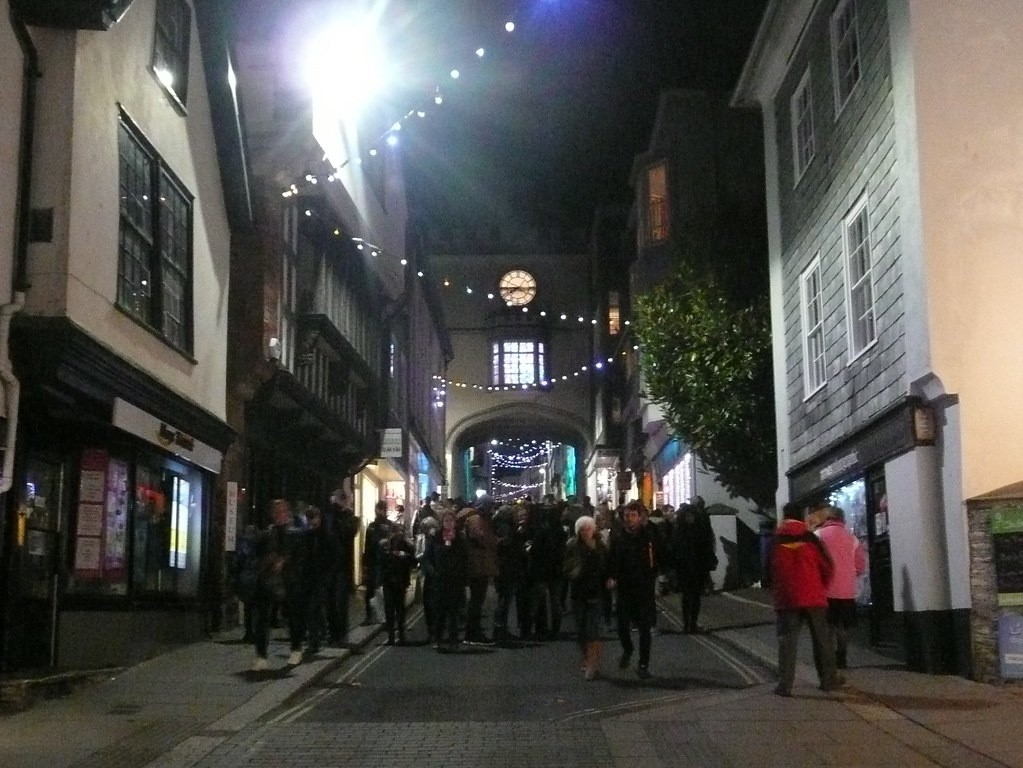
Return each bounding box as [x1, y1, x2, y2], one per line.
[581, 639, 603, 678]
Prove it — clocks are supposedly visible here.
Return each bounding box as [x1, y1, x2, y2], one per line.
[497, 269, 539, 308]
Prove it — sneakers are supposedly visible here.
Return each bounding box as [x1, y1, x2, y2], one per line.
[285, 649, 301, 667]
[819, 680, 842, 691]
[460, 633, 494, 647]
[252, 655, 270, 674]
[775, 685, 793, 696]
[638, 664, 652, 679]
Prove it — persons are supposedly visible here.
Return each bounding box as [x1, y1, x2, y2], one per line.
[562, 514, 616, 680]
[810, 507, 863, 676]
[764, 501, 836, 696]
[240, 491, 718, 672]
[610, 503, 665, 680]
